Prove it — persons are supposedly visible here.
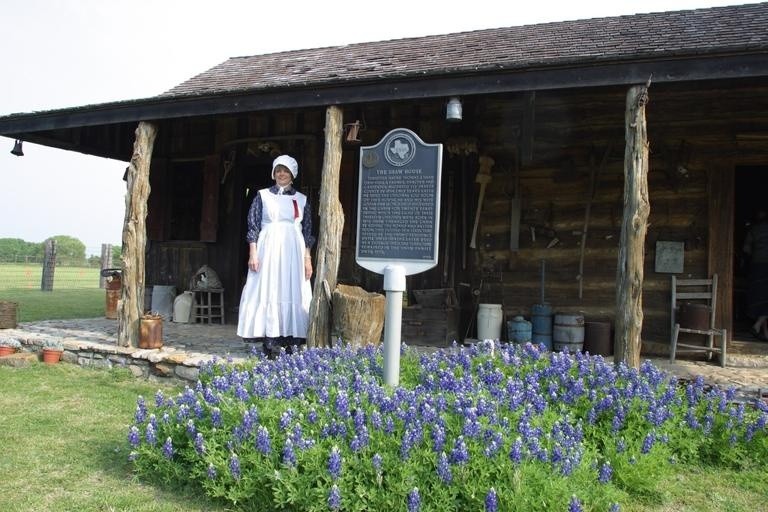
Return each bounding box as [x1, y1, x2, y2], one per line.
[236, 154, 317, 357]
[742, 210, 768, 342]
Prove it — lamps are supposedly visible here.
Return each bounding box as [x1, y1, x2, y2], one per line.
[445, 96, 462, 122]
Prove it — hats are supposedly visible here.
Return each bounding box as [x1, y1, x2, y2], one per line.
[271, 155, 299, 180]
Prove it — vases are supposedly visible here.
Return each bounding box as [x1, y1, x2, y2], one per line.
[42, 347, 62, 362]
[0, 344, 15, 357]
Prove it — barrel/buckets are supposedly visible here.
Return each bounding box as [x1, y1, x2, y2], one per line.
[531, 303, 554, 350]
[553, 312, 585, 353]
[477, 303, 503, 342]
[145, 285, 154, 312]
[173, 291, 198, 323]
[0, 302, 17, 329]
[506, 317, 533, 344]
[106, 289, 122, 320]
[151, 285, 177, 320]
[584, 319, 612, 357]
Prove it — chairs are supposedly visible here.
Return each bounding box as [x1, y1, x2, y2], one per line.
[668, 271, 729, 370]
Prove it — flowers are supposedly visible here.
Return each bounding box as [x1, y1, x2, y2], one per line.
[0, 334, 21, 348]
[40, 339, 64, 352]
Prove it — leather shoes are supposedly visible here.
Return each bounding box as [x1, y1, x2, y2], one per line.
[263, 344, 293, 359]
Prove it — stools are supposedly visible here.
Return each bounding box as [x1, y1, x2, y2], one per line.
[187, 288, 225, 325]
